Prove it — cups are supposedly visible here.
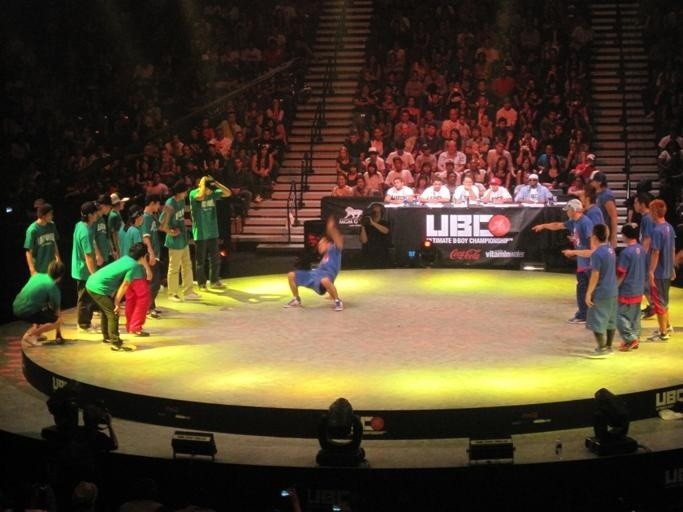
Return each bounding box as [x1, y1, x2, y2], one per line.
[552, 195, 557, 202]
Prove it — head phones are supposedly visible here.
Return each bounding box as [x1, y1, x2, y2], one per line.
[381, 204, 385, 217]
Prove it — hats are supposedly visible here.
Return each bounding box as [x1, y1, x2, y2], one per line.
[489, 177, 501, 185]
[81, 176, 217, 217]
[562, 154, 639, 238]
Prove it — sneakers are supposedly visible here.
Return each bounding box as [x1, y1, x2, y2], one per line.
[334, 299, 343, 311]
[284, 297, 301, 308]
[568, 305, 674, 358]
[23, 281, 227, 352]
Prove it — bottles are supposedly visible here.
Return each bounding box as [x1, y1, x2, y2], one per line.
[491, 195, 504, 204]
[403, 194, 422, 207]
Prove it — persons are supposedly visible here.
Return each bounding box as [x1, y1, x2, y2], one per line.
[331, 1, 596, 204]
[286, 216, 343, 311]
[637, 2, 683, 166]
[31, 379, 118, 472]
[2, 1, 320, 351]
[532, 169, 683, 352]
[113, 466, 371, 512]
[360, 202, 391, 269]
